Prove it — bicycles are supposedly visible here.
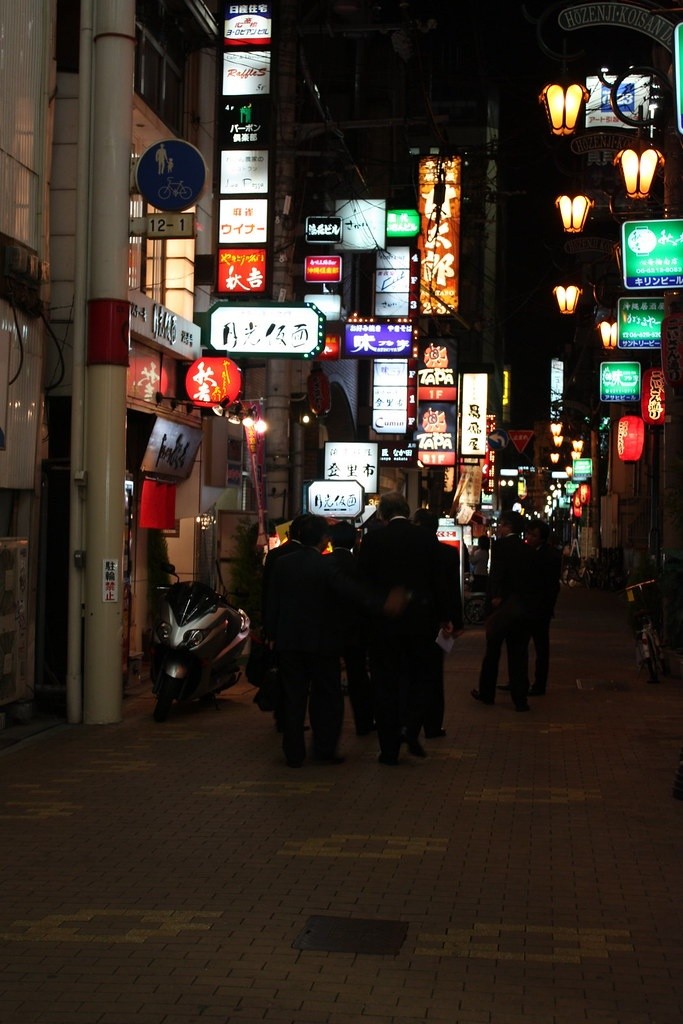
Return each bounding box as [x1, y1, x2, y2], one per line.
[616, 577, 670, 682]
[457, 571, 492, 626]
[561, 546, 634, 593]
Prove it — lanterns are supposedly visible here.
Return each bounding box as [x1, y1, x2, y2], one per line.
[573, 484, 591, 518]
[307, 367, 332, 419]
[641, 361, 665, 429]
[617, 410, 645, 464]
[660, 303, 683, 396]
[185, 349, 242, 416]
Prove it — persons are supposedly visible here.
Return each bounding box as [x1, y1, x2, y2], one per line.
[261, 494, 462, 768]
[470, 511, 562, 712]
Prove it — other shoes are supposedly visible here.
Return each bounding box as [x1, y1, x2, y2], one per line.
[513, 700, 530, 712]
[286, 755, 306, 767]
[425, 728, 446, 738]
[527, 686, 546, 696]
[408, 742, 426, 757]
[379, 754, 400, 765]
[472, 689, 495, 705]
[310, 752, 345, 765]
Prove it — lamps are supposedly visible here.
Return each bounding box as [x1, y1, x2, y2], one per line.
[298, 412, 318, 428]
[541, 412, 592, 483]
[613, 107, 669, 202]
[531, 61, 664, 141]
[151, 386, 280, 436]
[552, 274, 600, 319]
[592, 307, 619, 353]
[552, 183, 616, 241]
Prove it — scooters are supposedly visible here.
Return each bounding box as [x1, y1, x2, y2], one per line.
[149, 562, 252, 723]
